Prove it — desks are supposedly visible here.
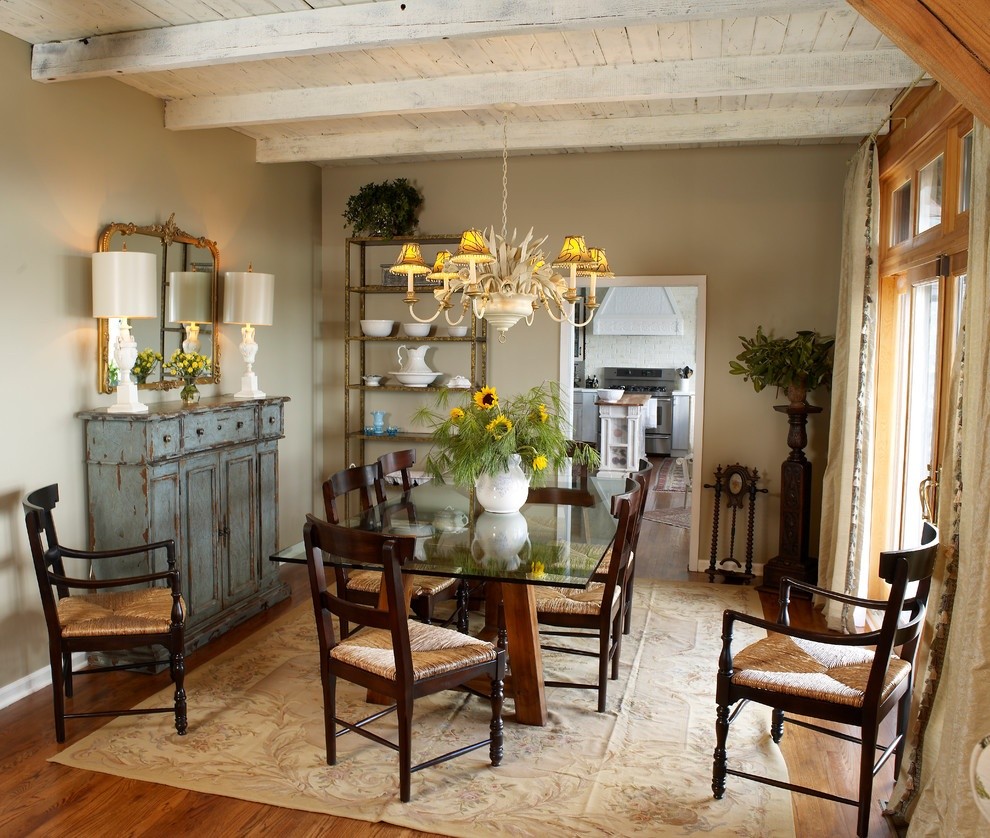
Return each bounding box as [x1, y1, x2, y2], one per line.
[593, 393, 651, 472]
[268, 467, 626, 637]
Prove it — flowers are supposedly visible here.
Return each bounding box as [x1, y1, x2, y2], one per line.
[407, 376, 604, 496]
[449, 542, 603, 585]
[161, 348, 212, 386]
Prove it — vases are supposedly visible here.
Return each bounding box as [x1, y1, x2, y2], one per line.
[474, 450, 529, 512]
[470, 509, 531, 573]
[181, 376, 200, 406]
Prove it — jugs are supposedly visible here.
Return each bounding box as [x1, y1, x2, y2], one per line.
[431, 505, 468, 532]
[397, 345, 431, 372]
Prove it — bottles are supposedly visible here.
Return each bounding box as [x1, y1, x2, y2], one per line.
[586, 375, 599, 388]
[574, 374, 581, 387]
[370, 411, 383, 433]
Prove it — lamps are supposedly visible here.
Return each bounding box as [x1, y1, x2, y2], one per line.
[90, 239, 158, 412]
[388, 99, 615, 346]
[165, 264, 212, 357]
[223, 261, 276, 398]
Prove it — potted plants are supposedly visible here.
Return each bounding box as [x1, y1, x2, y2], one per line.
[728, 325, 836, 403]
[341, 177, 425, 242]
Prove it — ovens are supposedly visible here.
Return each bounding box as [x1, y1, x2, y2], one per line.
[645, 396, 672, 434]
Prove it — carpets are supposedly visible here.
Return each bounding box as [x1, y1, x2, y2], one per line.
[653, 457, 691, 493]
[643, 505, 691, 530]
[45, 546, 797, 837]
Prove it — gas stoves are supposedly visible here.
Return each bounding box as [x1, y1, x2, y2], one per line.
[604, 368, 675, 396]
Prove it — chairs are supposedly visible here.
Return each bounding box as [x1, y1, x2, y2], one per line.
[303, 448, 653, 800]
[22, 482, 188, 740]
[710, 521, 941, 837]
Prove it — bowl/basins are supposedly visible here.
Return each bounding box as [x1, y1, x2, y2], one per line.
[597, 389, 625, 403]
[360, 320, 394, 336]
[447, 326, 467, 336]
[388, 371, 444, 387]
[402, 323, 431, 336]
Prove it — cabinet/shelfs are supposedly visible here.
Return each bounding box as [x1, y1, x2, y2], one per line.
[75, 394, 291, 674]
[344, 233, 489, 530]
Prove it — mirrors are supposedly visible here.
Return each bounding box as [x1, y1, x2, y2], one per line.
[96, 212, 220, 391]
[97, 390, 221, 404]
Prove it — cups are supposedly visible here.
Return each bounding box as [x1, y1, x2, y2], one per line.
[680, 379, 688, 391]
